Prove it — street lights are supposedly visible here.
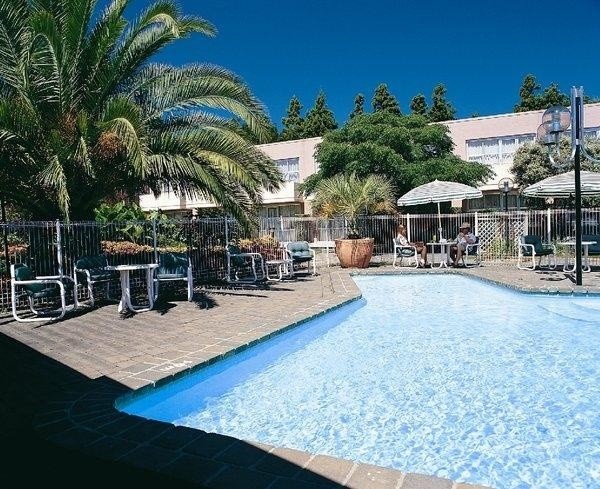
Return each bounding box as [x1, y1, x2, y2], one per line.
[536, 84, 599, 285]
[498, 178, 515, 255]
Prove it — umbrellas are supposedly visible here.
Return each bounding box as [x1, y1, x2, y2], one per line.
[397, 178, 483, 240]
[520, 170, 600, 198]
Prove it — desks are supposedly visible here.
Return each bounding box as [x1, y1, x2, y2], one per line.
[557, 240, 597, 273]
[426, 242, 457, 269]
[103, 264, 160, 314]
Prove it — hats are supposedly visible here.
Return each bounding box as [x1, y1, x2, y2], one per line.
[459, 221, 471, 229]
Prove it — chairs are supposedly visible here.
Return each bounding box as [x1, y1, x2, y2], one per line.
[446, 236, 481, 268]
[393, 238, 418, 269]
[74, 254, 122, 307]
[283, 241, 316, 278]
[226, 243, 266, 285]
[517, 235, 557, 271]
[580, 233, 600, 273]
[10, 263, 77, 322]
[153, 257, 193, 301]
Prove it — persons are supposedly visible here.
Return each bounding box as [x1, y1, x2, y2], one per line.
[397, 224, 431, 268]
[450, 222, 476, 267]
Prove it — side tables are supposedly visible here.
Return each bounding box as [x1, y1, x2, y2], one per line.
[265, 259, 293, 281]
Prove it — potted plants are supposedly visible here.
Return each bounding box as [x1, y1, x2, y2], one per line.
[308, 170, 397, 268]
